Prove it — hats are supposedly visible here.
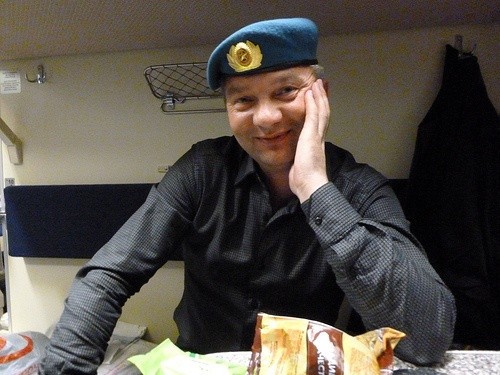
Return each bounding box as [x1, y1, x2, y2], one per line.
[206, 17, 319, 90]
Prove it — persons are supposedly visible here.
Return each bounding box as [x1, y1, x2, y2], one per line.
[37, 17, 457, 375]
